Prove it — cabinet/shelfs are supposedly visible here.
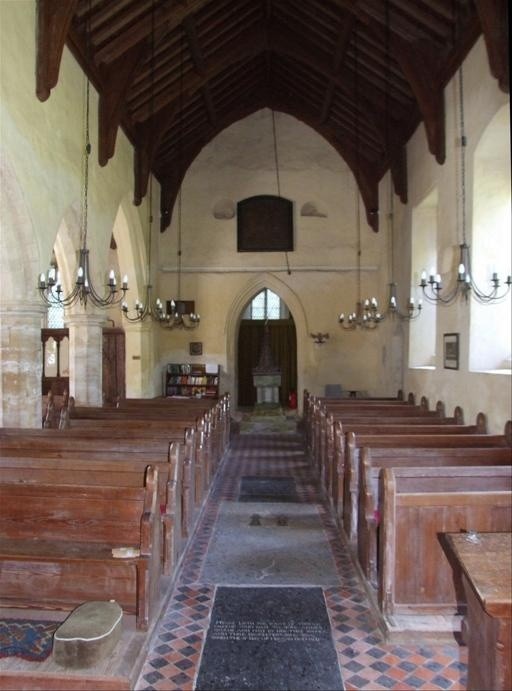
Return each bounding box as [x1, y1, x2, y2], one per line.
[166, 363, 220, 397]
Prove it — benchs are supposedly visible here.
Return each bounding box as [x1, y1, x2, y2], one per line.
[0, 392, 232, 632]
[302, 388, 512, 615]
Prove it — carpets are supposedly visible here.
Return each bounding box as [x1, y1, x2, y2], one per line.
[0, 618, 65, 663]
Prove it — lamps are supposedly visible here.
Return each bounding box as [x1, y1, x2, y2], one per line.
[338, 182, 381, 330]
[37, 73, 132, 311]
[117, 176, 178, 323]
[363, 177, 427, 326]
[156, 179, 200, 330]
[419, 63, 510, 308]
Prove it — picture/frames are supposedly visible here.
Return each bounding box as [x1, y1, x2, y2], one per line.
[443, 333, 460, 370]
[190, 342, 202, 355]
